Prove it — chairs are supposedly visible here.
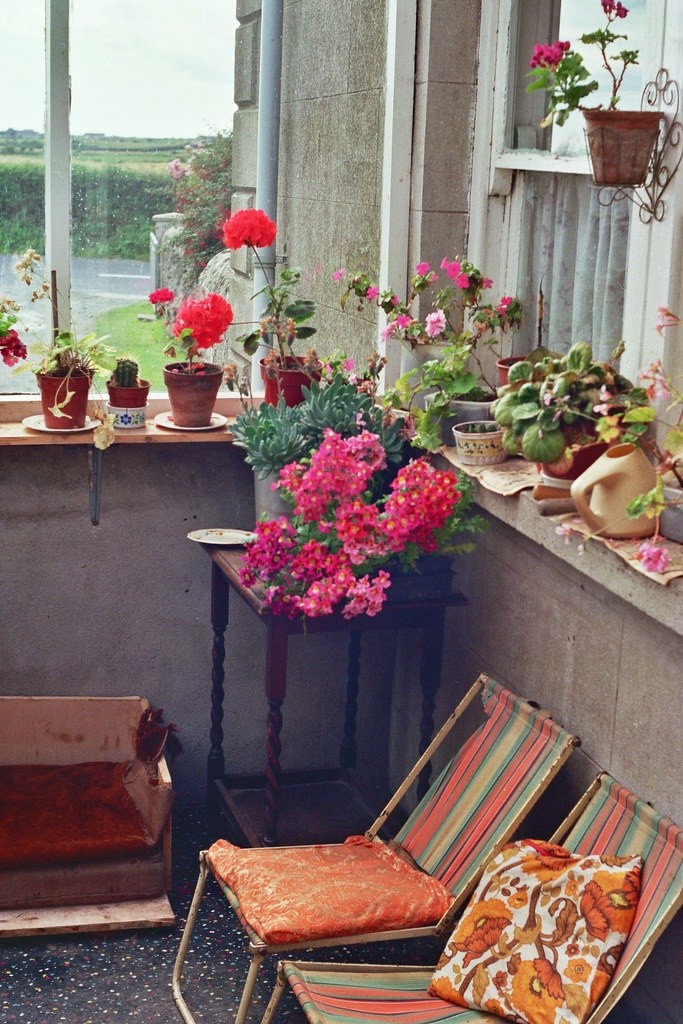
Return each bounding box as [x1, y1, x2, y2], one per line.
[253, 771, 682, 1023]
[172, 670, 583, 1022]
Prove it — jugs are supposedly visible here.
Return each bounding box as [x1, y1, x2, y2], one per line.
[570, 442, 662, 537]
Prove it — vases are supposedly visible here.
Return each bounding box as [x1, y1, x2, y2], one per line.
[259, 352, 317, 408]
[422, 384, 507, 450]
[585, 110, 667, 185]
[397, 338, 472, 419]
[650, 466, 681, 540]
[167, 360, 223, 427]
[376, 550, 454, 606]
[36, 365, 96, 430]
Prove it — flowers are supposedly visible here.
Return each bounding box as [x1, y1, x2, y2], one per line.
[0, 245, 106, 381]
[523, 1, 640, 131]
[237, 435, 460, 623]
[147, 289, 233, 375]
[542, 305, 682, 572]
[322, 255, 511, 432]
[219, 208, 321, 376]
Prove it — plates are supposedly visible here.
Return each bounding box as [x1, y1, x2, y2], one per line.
[186, 529, 255, 544]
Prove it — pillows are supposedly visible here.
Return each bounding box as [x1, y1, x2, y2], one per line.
[427, 837, 648, 1022]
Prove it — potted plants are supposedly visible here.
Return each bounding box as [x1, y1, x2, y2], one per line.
[454, 420, 507, 465]
[497, 270, 546, 388]
[507, 338, 646, 484]
[107, 355, 148, 430]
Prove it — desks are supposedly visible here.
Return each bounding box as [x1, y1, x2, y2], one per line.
[199, 540, 470, 847]
